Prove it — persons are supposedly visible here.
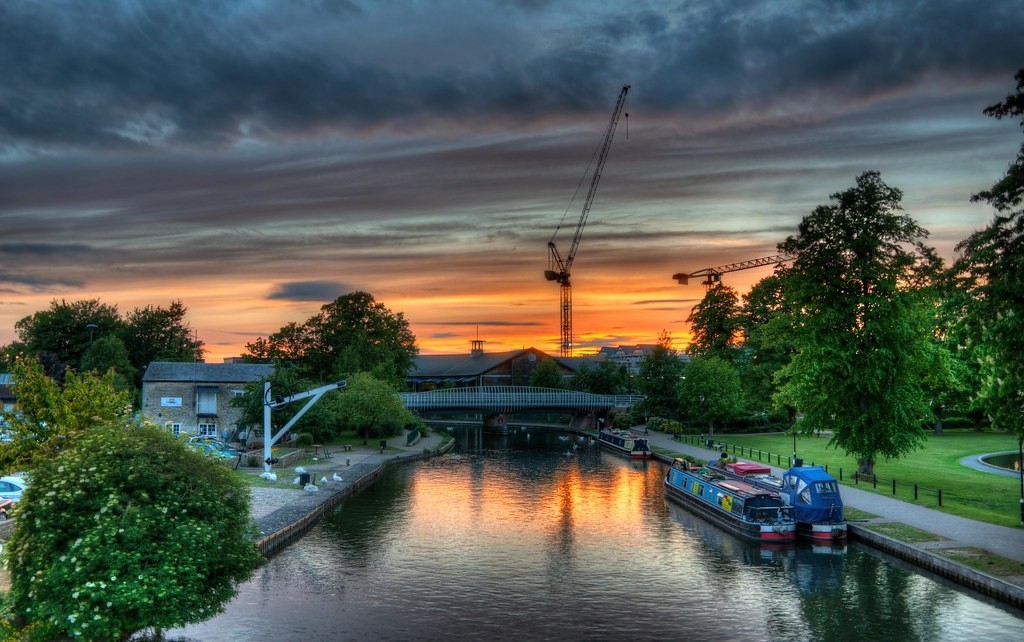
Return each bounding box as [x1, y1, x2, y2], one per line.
[221, 427, 247, 452]
[644, 425, 648, 436]
[558, 415, 570, 424]
[286, 431, 298, 444]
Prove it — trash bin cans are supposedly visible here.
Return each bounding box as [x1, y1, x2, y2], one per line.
[707, 439, 714, 447]
[380, 440, 386, 449]
[795, 459, 803, 467]
[300, 473, 310, 487]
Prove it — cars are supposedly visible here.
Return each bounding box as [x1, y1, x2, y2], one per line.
[0, 497, 16, 522]
[0, 472, 32, 503]
[183, 435, 240, 459]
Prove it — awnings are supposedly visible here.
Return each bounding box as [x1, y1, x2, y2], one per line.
[196, 386, 219, 393]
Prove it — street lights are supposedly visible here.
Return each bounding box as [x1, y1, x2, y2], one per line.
[86, 323, 98, 371]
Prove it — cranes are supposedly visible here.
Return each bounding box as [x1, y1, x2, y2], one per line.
[671, 253, 797, 346]
[545, 84, 631, 359]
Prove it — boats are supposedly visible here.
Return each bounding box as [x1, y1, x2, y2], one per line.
[706, 459, 847, 540]
[664, 458, 797, 542]
[596, 426, 652, 459]
[481, 413, 513, 436]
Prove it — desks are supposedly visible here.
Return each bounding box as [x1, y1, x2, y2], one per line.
[312, 444, 323, 455]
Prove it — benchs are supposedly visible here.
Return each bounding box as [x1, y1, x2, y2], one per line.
[343, 444, 352, 452]
[714, 444, 724, 452]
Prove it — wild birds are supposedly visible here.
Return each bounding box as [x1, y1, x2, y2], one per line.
[295, 467, 307, 474]
[303, 483, 319, 495]
[321, 477, 327, 484]
[270, 472, 277, 481]
[333, 473, 343, 485]
